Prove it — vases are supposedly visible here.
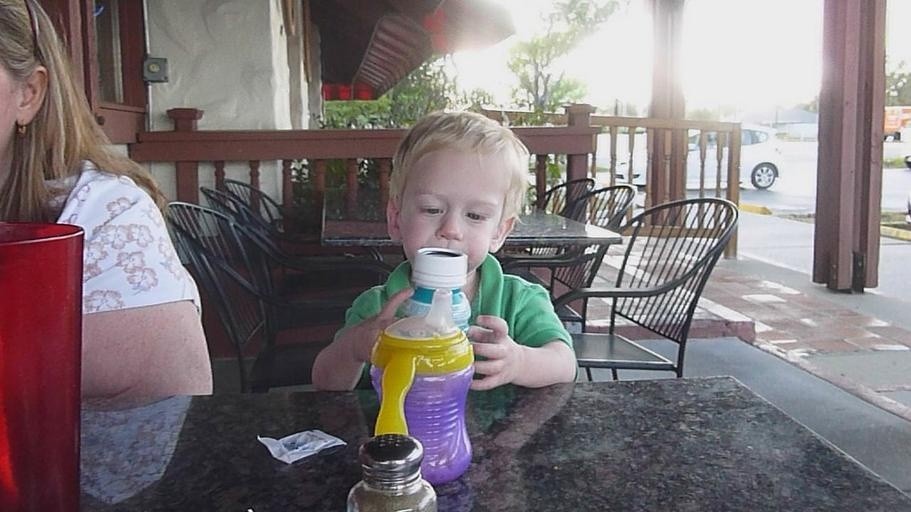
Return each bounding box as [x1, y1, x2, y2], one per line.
[1, 220, 86, 510]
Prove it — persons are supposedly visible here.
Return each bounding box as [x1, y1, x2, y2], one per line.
[0, 1, 214, 403]
[311, 110, 579, 392]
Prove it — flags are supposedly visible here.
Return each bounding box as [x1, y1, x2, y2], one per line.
[1, 220, 86, 510]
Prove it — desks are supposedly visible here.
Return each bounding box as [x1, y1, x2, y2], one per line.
[70, 376, 908, 512]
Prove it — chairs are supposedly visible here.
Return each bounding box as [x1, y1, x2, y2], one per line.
[163, 175, 743, 394]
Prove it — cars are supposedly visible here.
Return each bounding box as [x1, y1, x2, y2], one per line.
[1, 221, 83, 512]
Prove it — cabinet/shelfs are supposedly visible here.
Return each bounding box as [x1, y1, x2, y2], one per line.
[370, 291, 475, 485]
[347, 433, 440, 511]
[397, 246, 473, 332]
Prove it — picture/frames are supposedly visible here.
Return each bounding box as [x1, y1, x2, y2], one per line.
[70, 376, 908, 512]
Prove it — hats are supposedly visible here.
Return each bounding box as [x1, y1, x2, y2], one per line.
[616, 124, 783, 190]
[883, 107, 911, 141]
